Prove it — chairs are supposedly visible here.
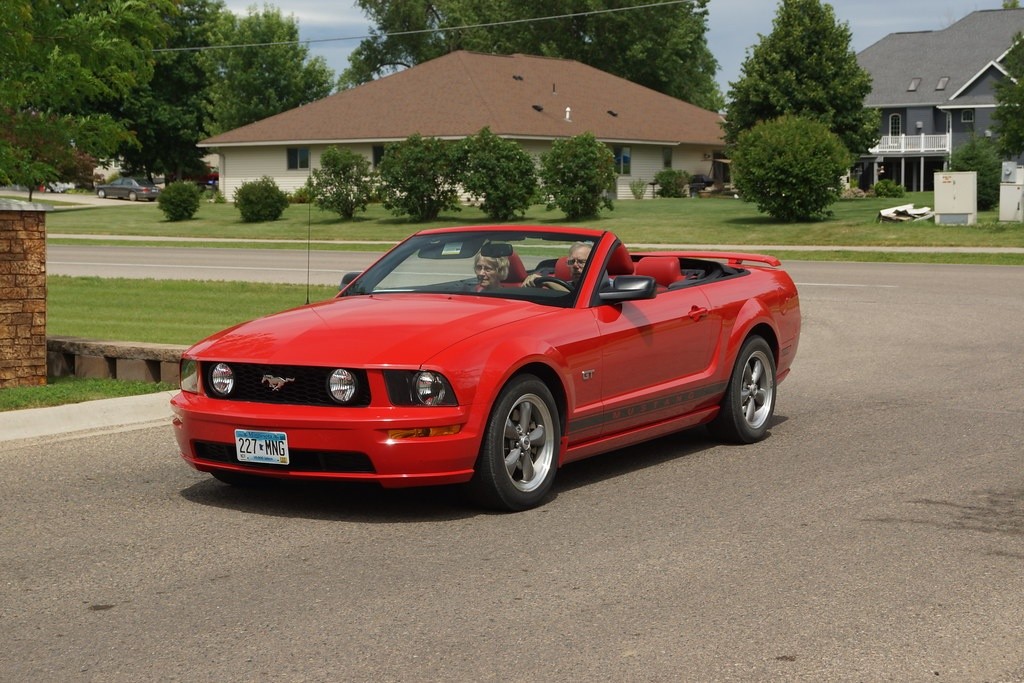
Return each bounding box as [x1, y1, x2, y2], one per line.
[474, 251, 531, 291]
[541, 255, 573, 290]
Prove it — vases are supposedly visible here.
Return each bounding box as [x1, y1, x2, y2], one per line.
[698, 190, 712, 198]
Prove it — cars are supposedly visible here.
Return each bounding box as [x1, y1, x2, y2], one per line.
[688, 174, 715, 198]
[95, 177, 160, 202]
[167, 166, 219, 186]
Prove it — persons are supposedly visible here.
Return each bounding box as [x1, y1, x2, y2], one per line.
[473, 249, 510, 294]
[520, 242, 616, 294]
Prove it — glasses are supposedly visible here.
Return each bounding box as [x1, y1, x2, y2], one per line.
[568, 259, 586, 265]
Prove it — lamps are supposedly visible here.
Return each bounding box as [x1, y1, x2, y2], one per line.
[916, 121, 924, 128]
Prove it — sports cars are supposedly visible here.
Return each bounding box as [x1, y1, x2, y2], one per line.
[170, 224, 801, 512]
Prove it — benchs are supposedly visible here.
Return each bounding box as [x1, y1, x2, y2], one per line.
[607, 257, 689, 293]
